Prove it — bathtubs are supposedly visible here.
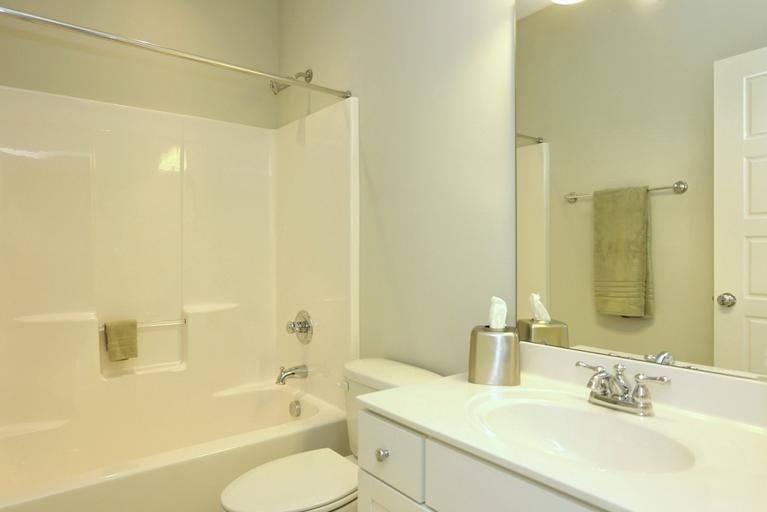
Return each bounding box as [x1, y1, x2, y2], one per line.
[1, 379, 352, 512]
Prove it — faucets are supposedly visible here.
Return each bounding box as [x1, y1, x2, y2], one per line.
[655, 352, 675, 366]
[275, 365, 308, 385]
[585, 372, 627, 401]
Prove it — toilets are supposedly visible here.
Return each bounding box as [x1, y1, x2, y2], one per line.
[219, 357, 446, 512]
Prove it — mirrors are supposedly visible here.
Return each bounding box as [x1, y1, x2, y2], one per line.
[513, 0, 767, 385]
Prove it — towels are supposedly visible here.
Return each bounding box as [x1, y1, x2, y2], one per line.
[589, 184, 654, 322]
[105, 318, 138, 363]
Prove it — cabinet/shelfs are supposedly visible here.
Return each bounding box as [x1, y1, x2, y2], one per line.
[353, 404, 614, 512]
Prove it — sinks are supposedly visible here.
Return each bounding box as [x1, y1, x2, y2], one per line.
[465, 389, 697, 475]
